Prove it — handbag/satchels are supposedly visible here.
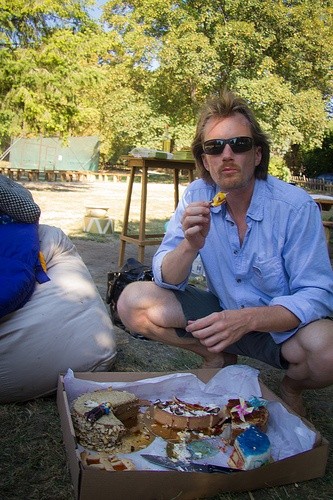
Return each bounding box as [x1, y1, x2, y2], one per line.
[105, 256, 155, 341]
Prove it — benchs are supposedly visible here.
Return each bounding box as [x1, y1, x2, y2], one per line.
[0, 166, 150, 184]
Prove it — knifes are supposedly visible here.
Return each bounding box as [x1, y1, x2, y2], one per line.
[140, 454, 246, 475]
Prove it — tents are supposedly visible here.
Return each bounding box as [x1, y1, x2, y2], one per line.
[8, 136, 99, 172]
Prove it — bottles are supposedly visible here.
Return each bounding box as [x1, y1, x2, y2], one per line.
[233, 425, 271, 472]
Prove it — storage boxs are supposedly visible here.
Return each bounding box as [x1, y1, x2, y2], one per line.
[57, 364, 331, 500]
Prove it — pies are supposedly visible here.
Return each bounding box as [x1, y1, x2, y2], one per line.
[80, 452, 137, 472]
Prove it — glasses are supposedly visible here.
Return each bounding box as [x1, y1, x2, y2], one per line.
[194, 137, 257, 156]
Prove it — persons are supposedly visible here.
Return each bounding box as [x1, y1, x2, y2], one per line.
[118, 95, 333, 423]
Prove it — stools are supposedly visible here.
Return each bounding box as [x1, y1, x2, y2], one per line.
[118, 152, 196, 269]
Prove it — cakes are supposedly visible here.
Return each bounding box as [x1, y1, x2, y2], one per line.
[227, 425, 270, 471]
[225, 396, 269, 442]
[209, 191, 228, 207]
[71, 388, 140, 449]
[152, 397, 220, 431]
